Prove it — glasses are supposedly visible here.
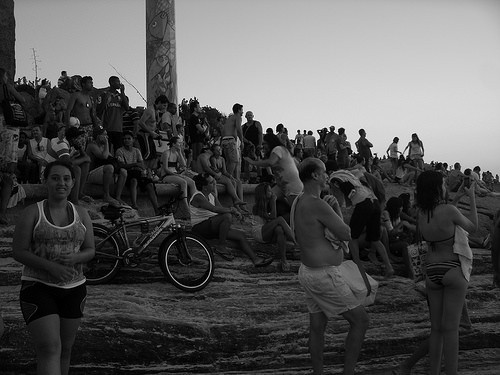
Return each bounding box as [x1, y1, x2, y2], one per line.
[37, 143, 40, 151]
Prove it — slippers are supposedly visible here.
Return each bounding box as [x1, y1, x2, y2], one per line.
[213, 249, 235, 261]
[255, 256, 275, 268]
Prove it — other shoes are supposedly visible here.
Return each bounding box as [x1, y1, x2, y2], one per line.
[481, 234, 493, 250]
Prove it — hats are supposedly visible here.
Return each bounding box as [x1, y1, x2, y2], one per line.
[65, 127, 85, 140]
[92, 125, 107, 136]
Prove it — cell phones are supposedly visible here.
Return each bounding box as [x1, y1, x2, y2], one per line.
[464, 177, 470, 189]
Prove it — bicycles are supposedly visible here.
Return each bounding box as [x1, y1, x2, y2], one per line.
[83, 194, 215, 294]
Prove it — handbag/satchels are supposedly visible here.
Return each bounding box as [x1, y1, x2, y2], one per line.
[407, 215, 429, 283]
[1, 83, 29, 126]
[338, 256, 379, 307]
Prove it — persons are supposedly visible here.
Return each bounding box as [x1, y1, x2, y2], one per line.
[12, 161, 96, 375]
[392, 291, 471, 375]
[0, 68, 500, 289]
[292, 158, 369, 375]
[416, 170, 478, 375]
